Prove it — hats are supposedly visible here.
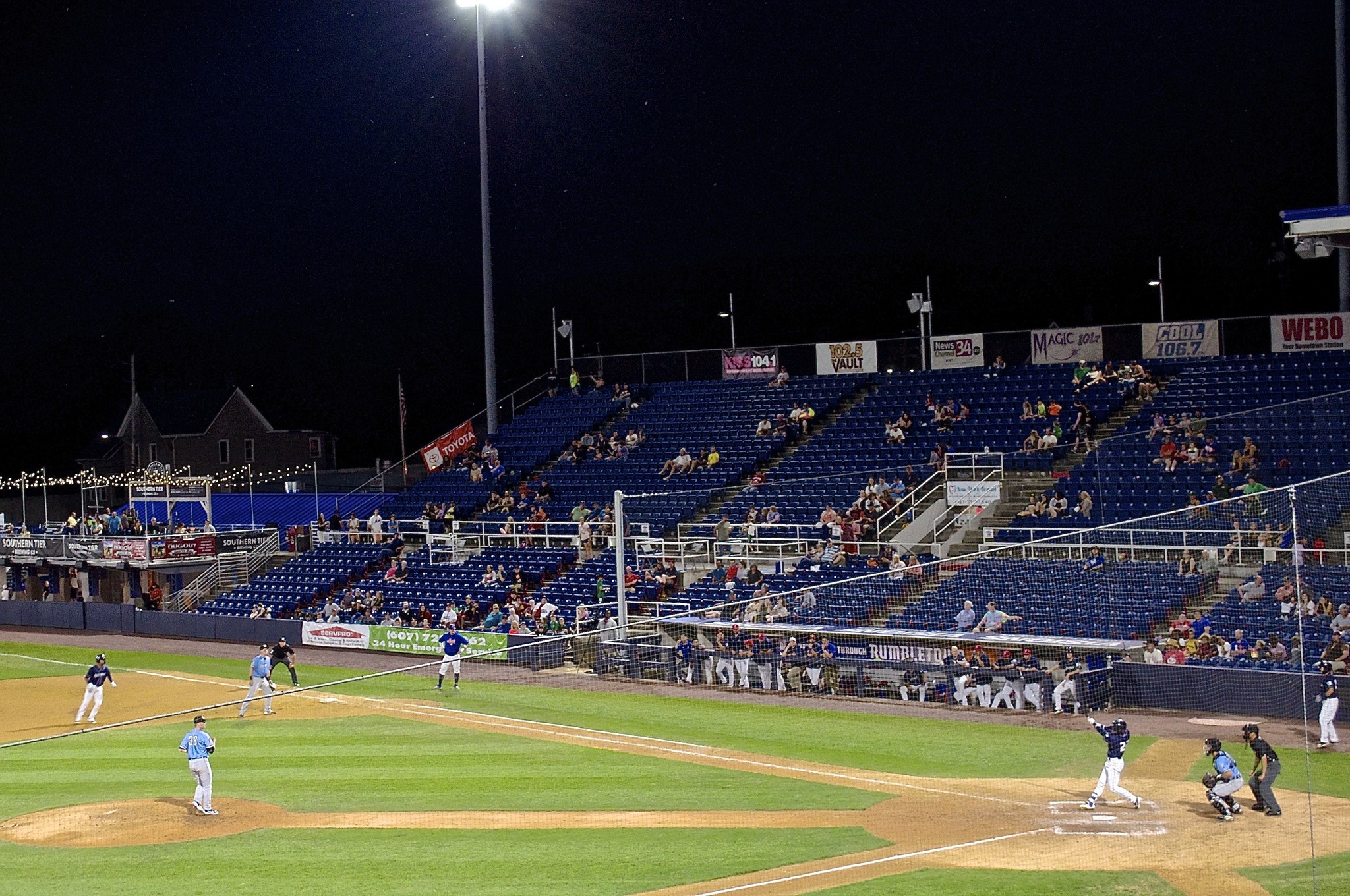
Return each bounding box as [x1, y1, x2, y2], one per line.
[493, 595, 1074, 658]
[193, 715, 206, 723]
[278, 637, 286, 643]
[260, 645, 269, 651]
[448, 623, 456, 629]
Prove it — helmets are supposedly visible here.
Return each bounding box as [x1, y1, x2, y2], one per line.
[1203, 737, 1221, 756]
[95, 654, 106, 663]
[1320, 660, 1332, 674]
[1241, 723, 1258, 740]
[1112, 719, 1127, 731]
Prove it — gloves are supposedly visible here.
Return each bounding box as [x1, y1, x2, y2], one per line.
[87, 683, 93, 693]
[1315, 693, 1323, 703]
[1088, 718, 1102, 728]
[111, 681, 117, 688]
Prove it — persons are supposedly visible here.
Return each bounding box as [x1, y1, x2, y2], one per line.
[249, 588, 481, 630]
[1201, 738, 1244, 821]
[178, 716, 218, 815]
[486, 478, 629, 561]
[148, 581, 162, 611]
[1314, 662, 1340, 748]
[1, 568, 84, 601]
[1080, 717, 1141, 810]
[318, 500, 459, 584]
[433, 624, 469, 689]
[5, 508, 216, 538]
[535, 366, 632, 410]
[564, 430, 645, 460]
[478, 560, 679, 672]
[269, 637, 300, 686]
[1241, 724, 1282, 816]
[74, 653, 116, 724]
[657, 356, 1161, 634]
[441, 439, 505, 493]
[675, 624, 1081, 717]
[239, 644, 277, 717]
[1122, 414, 1350, 677]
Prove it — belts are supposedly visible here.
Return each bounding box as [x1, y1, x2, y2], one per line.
[189, 756, 208, 760]
[257, 676, 265, 678]
[1231, 775, 1241, 780]
[1109, 757, 1122, 760]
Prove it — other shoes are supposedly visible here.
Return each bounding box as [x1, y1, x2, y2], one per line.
[264, 711, 276, 715]
[294, 682, 300, 687]
[240, 714, 244, 717]
[1215, 804, 1282, 821]
[74, 717, 97, 724]
[455, 686, 460, 690]
[434, 686, 443, 689]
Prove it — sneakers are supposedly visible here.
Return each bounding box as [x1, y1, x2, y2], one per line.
[1316, 742, 1340, 749]
[1133, 796, 1141, 809]
[1079, 803, 1095, 810]
[191, 801, 219, 815]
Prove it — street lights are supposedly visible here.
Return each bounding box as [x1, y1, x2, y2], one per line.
[718, 292, 735, 350]
[1148, 256, 1165, 322]
[454, 1, 513, 432]
[101, 433, 138, 472]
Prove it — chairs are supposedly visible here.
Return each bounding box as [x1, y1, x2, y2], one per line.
[186, 353, 1349, 724]
